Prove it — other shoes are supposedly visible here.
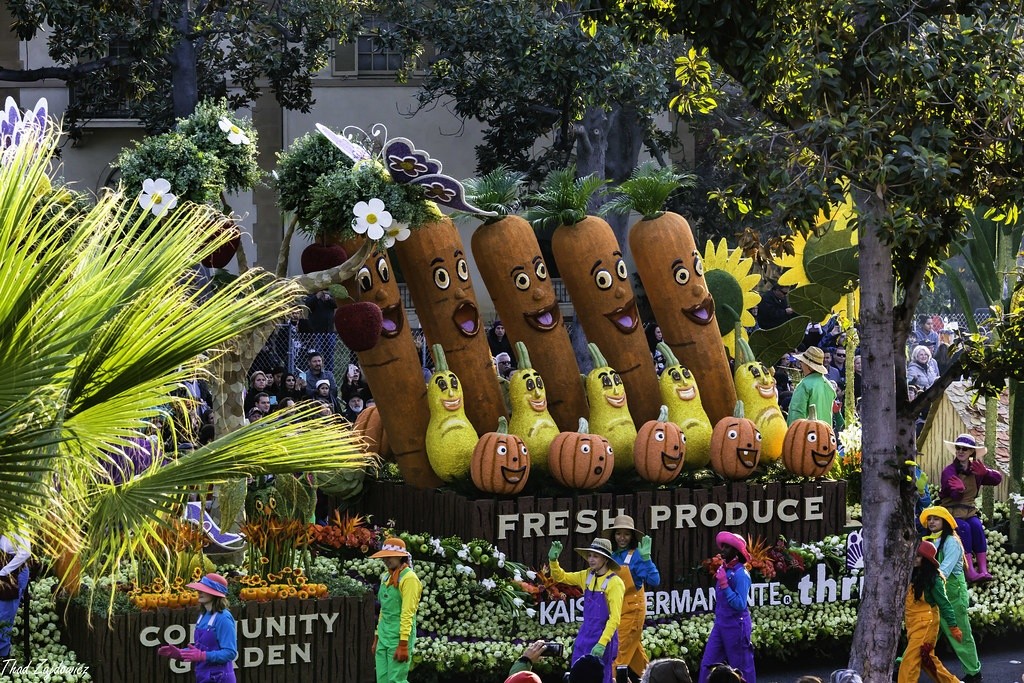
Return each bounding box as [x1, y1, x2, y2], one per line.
[961, 672, 983, 683]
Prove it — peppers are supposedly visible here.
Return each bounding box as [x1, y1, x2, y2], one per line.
[241, 583, 327, 600]
[134, 593, 199, 607]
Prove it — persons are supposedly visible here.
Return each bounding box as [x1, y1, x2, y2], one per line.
[421, 315, 1003, 683]
[0, 354, 374, 683]
[367, 537, 424, 683]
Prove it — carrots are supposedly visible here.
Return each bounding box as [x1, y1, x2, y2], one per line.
[312, 208, 740, 485]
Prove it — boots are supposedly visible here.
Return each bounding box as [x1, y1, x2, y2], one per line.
[964, 551, 992, 582]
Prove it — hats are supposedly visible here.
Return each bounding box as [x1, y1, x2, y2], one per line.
[792, 346, 828, 374]
[369, 538, 410, 559]
[716, 531, 750, 560]
[573, 537, 621, 571]
[316, 380, 330, 390]
[186, 572, 227, 597]
[943, 433, 987, 458]
[919, 505, 961, 530]
[569, 654, 604, 683]
[919, 339, 937, 346]
[249, 407, 262, 415]
[602, 515, 645, 541]
[918, 541, 941, 568]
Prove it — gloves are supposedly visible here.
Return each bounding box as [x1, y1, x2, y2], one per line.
[591, 644, 606, 657]
[547, 540, 563, 560]
[372, 636, 378, 655]
[716, 566, 729, 589]
[393, 640, 409, 662]
[157, 642, 206, 663]
[948, 460, 988, 491]
[637, 535, 652, 560]
[949, 627, 963, 643]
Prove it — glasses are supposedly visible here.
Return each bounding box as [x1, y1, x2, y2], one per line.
[498, 361, 511, 365]
[955, 446, 968, 451]
[836, 353, 846, 358]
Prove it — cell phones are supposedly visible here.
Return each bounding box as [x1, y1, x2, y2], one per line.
[539, 643, 564, 657]
[616, 665, 628, 683]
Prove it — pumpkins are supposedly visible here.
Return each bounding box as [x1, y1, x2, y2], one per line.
[347, 341, 837, 491]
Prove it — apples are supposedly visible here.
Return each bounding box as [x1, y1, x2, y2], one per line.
[400, 532, 490, 563]
[801, 534, 848, 567]
